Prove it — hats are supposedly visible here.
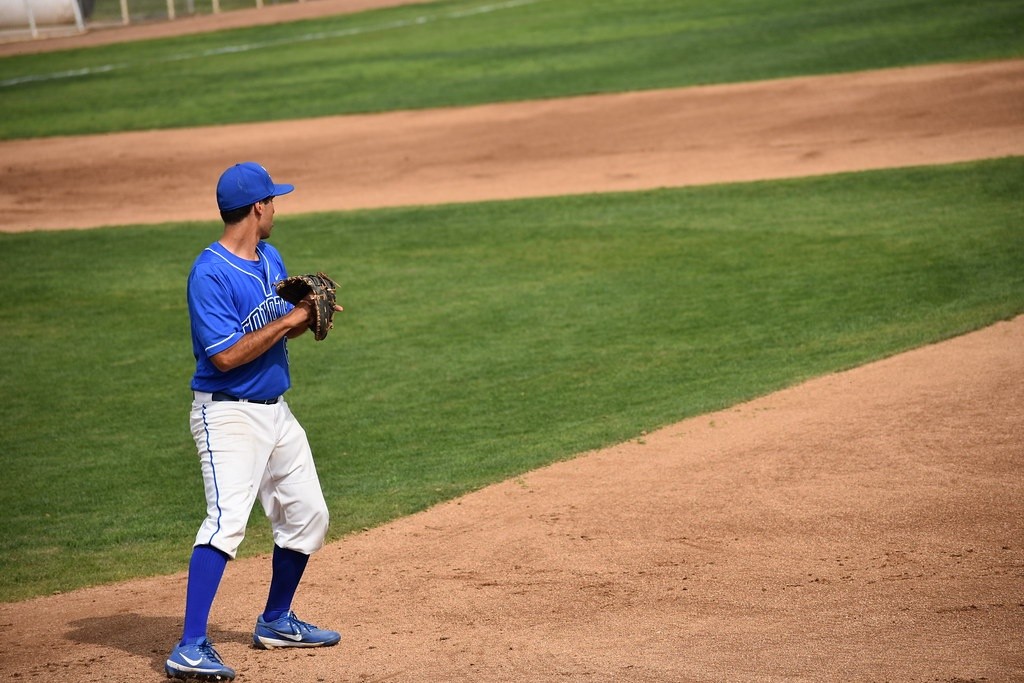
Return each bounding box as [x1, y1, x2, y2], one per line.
[216, 161, 294, 214]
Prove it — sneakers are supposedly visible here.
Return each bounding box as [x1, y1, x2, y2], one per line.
[165, 636, 235, 682]
[253, 610, 341, 650]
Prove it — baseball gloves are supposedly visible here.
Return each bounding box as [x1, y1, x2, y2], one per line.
[273, 271, 338, 341]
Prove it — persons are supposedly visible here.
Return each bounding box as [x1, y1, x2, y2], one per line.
[158, 161, 346, 676]
